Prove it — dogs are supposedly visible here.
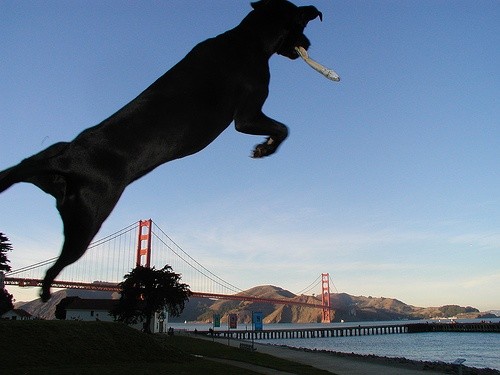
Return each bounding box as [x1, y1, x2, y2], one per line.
[0, 1, 324, 302]
